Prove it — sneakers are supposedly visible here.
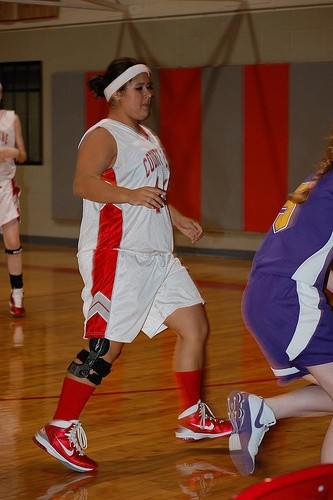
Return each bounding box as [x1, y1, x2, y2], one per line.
[227, 391, 276, 477]
[33, 420, 99, 473]
[9, 289, 26, 317]
[173, 403, 234, 443]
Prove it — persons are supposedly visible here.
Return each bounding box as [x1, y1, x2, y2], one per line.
[0, 83, 27, 316]
[228, 137, 333, 477]
[34, 459, 239, 500]
[32, 57, 235, 472]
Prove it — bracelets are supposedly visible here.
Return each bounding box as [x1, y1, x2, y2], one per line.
[16, 149, 19, 161]
